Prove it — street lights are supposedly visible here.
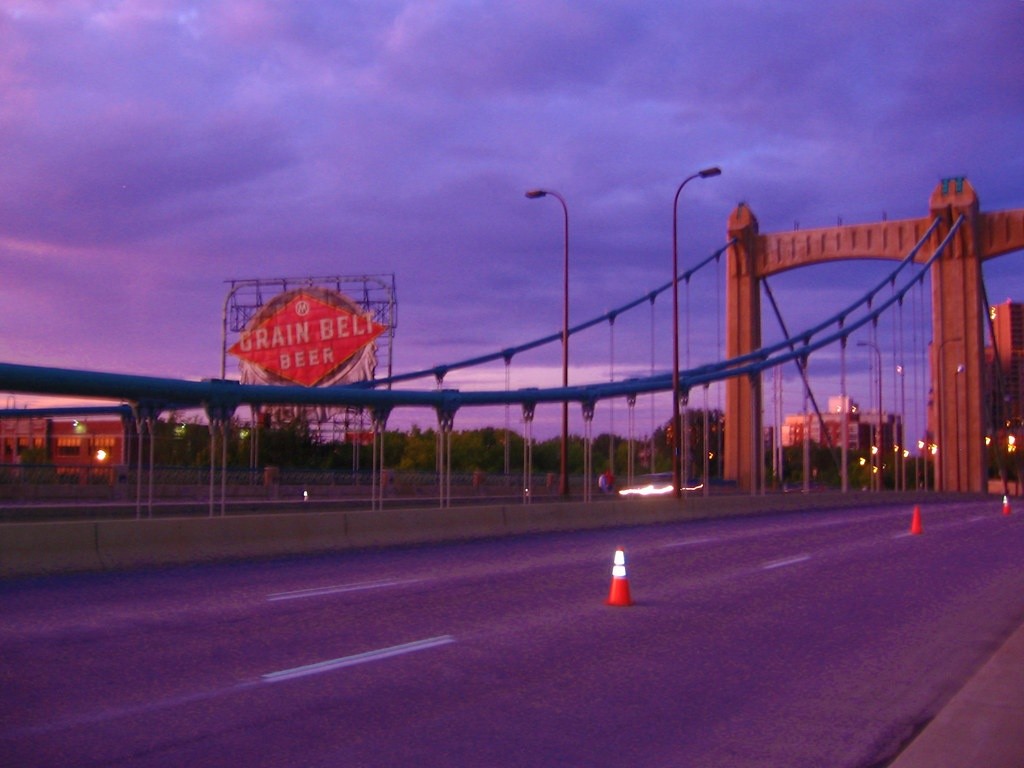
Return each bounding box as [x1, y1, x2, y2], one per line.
[524, 186, 572, 501]
[857, 341, 884, 494]
[671, 163, 725, 501]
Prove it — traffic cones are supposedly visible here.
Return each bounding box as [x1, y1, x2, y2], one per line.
[1002, 494, 1011, 516]
[909, 504, 923, 535]
[601, 544, 638, 607]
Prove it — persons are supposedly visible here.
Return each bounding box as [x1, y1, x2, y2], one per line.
[598, 469, 614, 494]
